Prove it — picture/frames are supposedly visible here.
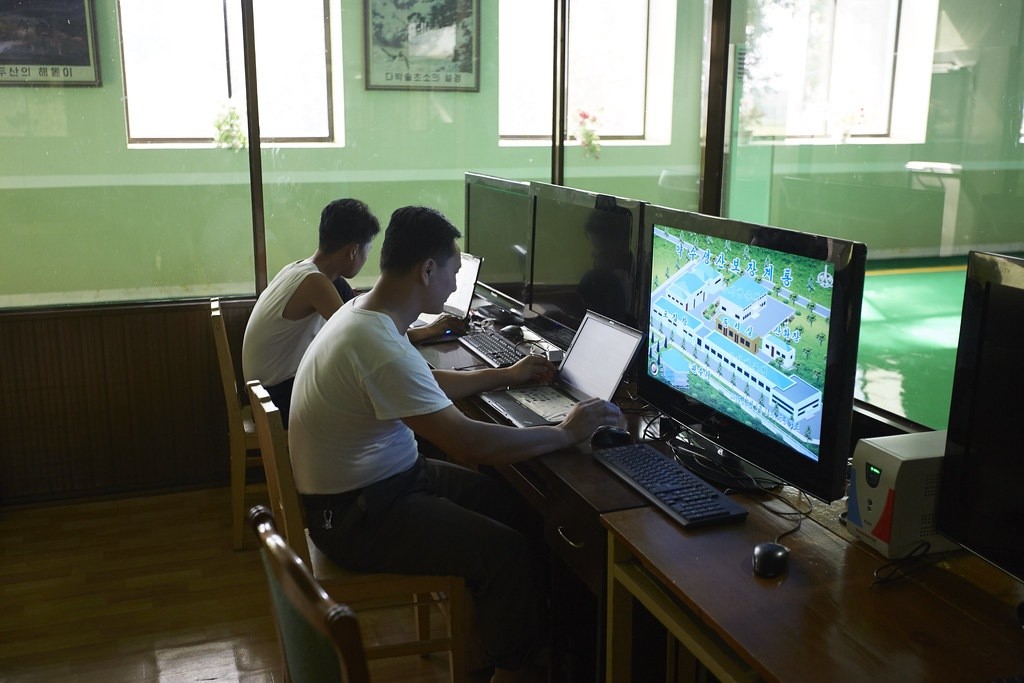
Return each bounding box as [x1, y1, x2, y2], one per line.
[0, 0, 107, 88]
[365, 0, 483, 94]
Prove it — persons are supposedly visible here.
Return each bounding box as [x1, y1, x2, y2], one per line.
[289, 204, 626, 683]
[242, 198, 468, 427]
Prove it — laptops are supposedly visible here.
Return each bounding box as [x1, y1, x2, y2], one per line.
[479, 308, 645, 429]
[409, 253, 479, 330]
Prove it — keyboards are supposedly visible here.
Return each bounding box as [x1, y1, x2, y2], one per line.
[458, 331, 525, 367]
[593, 443, 749, 528]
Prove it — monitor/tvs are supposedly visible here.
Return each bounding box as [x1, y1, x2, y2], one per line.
[462, 172, 868, 504]
[931, 250, 1024, 591]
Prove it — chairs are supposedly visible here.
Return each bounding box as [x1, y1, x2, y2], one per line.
[241, 381, 471, 683]
[207, 294, 293, 551]
[254, 521, 368, 683]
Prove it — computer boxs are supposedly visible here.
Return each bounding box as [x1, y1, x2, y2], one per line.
[845, 428, 965, 558]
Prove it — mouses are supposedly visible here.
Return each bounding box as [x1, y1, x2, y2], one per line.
[751, 542, 791, 579]
[590, 425, 635, 448]
[500, 325, 524, 338]
[445, 329, 454, 335]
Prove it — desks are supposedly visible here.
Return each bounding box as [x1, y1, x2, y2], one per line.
[410, 301, 1024, 683]
